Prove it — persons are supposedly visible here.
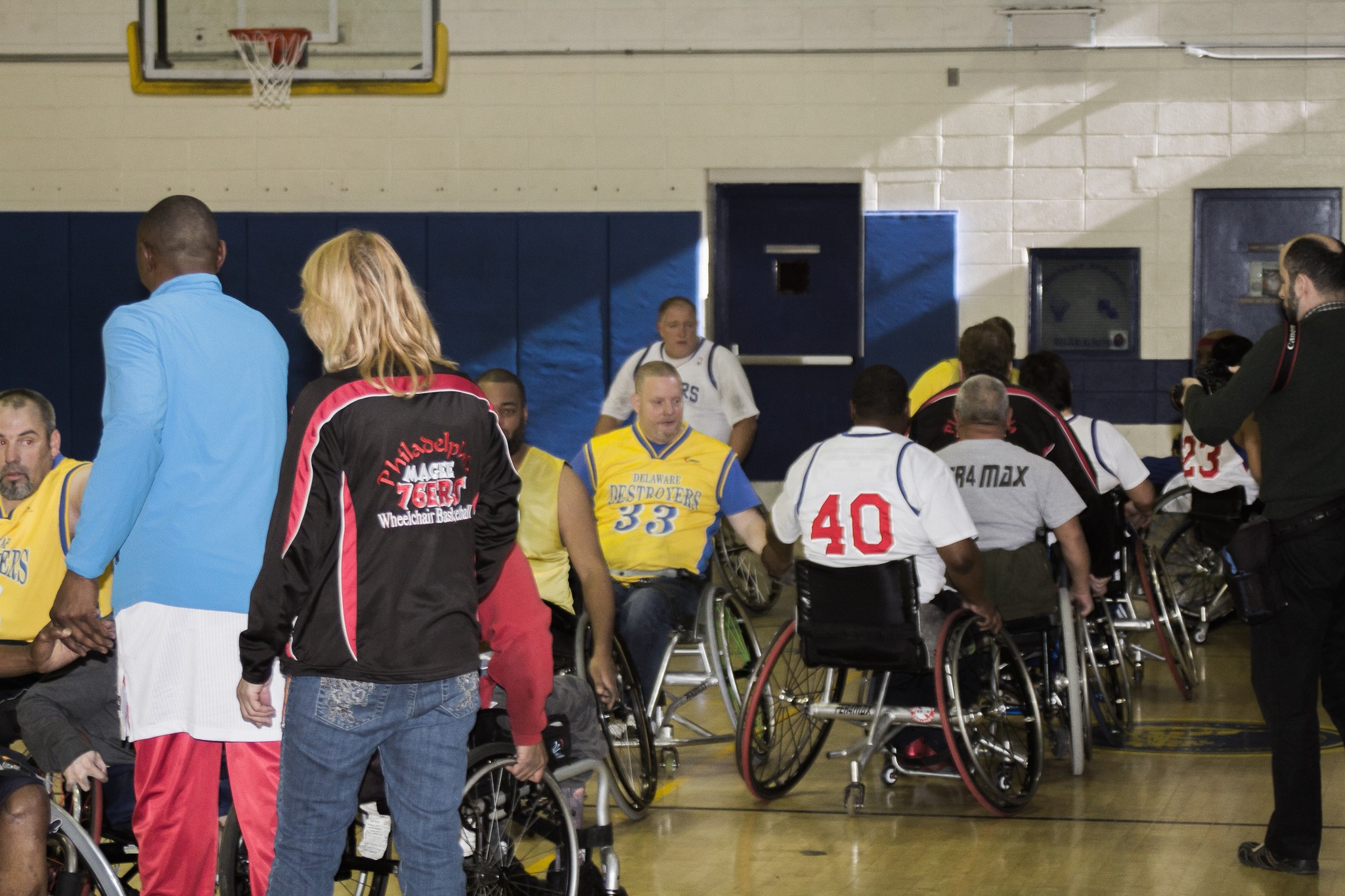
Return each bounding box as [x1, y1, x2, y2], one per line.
[473, 368, 621, 710]
[565, 362, 789, 732]
[473, 539, 552, 783]
[1178, 230, 1345, 875]
[1195, 328, 1235, 367]
[1180, 333, 1263, 590]
[591, 296, 762, 466]
[9, 644, 233, 831]
[236, 229, 519, 896]
[47, 195, 288, 896]
[0, 387, 130, 895]
[768, 311, 1153, 784]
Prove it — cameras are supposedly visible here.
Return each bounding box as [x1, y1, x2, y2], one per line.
[1171, 364, 1234, 412]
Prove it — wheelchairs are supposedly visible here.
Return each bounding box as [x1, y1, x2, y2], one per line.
[731, 573, 1045, 819]
[0, 457, 1247, 896]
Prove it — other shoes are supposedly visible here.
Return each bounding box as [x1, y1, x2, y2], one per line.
[1238, 840, 1319, 874]
